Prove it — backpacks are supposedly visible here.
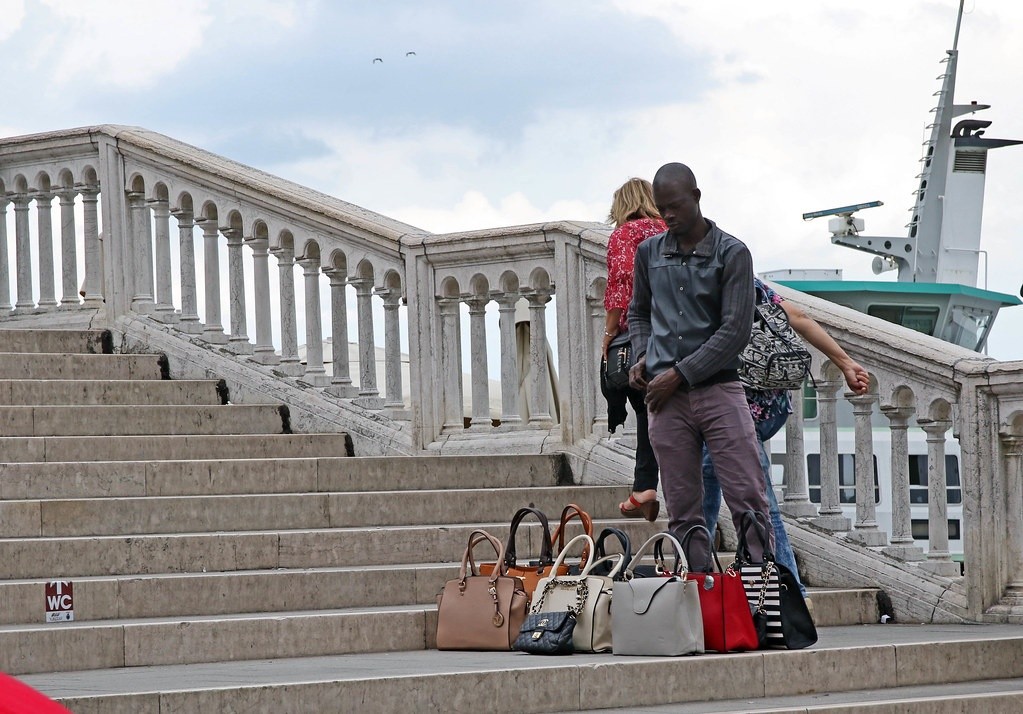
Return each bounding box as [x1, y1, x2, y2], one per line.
[735, 287, 811, 390]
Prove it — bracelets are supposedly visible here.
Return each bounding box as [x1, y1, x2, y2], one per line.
[604, 327, 616, 336]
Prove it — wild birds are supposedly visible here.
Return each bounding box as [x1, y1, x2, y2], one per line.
[406, 51, 416, 56]
[373, 58, 383, 64]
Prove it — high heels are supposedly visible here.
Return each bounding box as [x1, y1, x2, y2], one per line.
[620, 495, 659, 522]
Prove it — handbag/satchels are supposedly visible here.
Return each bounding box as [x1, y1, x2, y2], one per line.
[435, 497, 818, 656]
[602, 328, 636, 389]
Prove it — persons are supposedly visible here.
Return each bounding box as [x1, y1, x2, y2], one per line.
[629, 163, 775, 573]
[601, 178, 672, 522]
[699, 278, 871, 606]
